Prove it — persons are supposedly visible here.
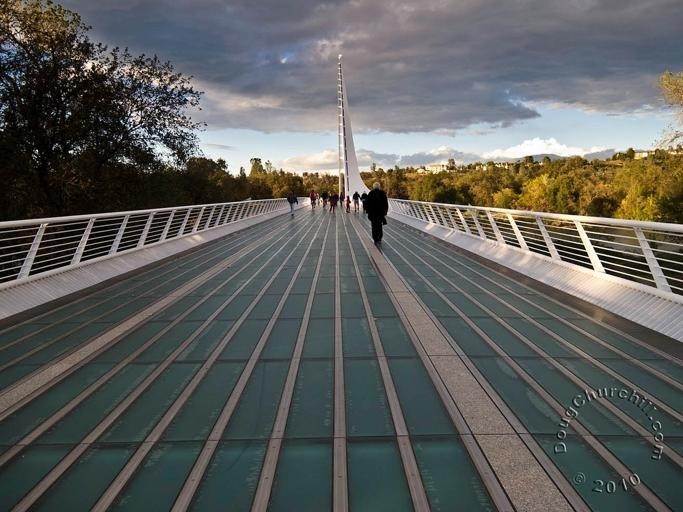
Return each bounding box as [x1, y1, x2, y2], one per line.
[308, 189, 367, 214]
[286, 192, 298, 216]
[363, 182, 389, 248]
